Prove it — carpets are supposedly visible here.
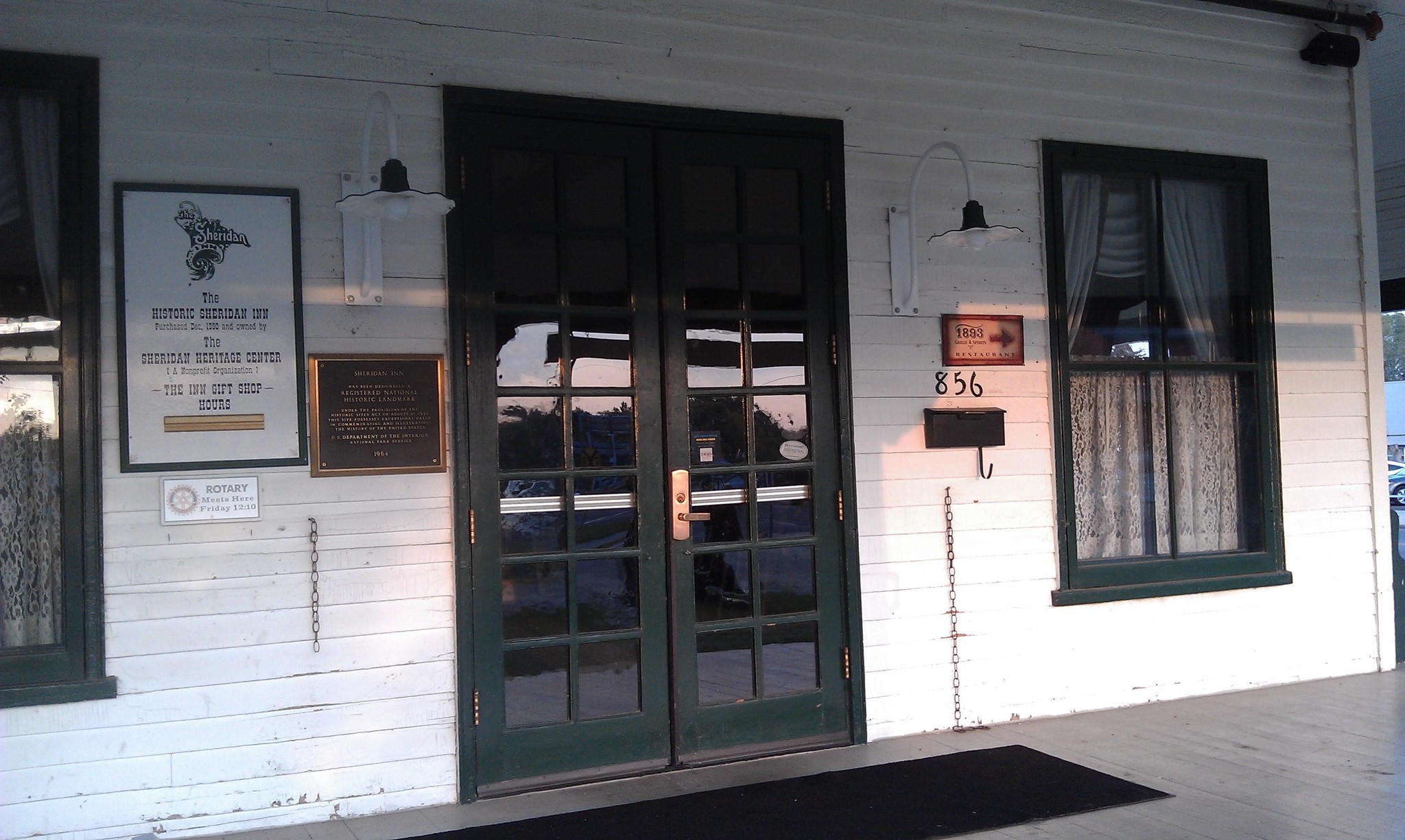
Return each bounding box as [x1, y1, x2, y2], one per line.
[391, 742, 1176, 839]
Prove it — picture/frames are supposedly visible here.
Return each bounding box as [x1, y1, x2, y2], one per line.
[112, 180, 310, 475]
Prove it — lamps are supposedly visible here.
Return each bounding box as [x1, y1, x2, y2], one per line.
[332, 91, 459, 308]
[886, 141, 1023, 317]
